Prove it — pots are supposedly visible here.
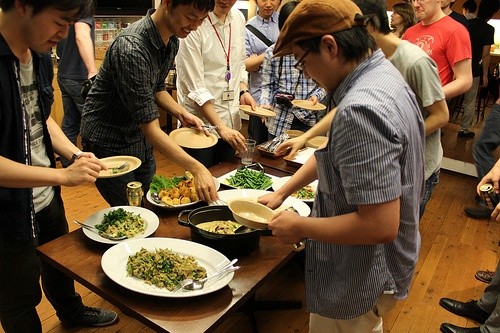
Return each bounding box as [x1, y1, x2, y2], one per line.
[178, 204, 261, 257]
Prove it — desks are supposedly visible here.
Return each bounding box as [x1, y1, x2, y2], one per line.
[34, 157, 312, 333]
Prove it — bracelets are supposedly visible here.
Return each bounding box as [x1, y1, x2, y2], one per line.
[70, 151, 83, 164]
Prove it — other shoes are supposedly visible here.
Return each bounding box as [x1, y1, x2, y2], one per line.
[458, 129, 475, 141]
[465, 208, 493, 219]
[383, 284, 395, 295]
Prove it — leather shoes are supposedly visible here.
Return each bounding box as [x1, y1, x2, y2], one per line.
[441, 323, 481, 333]
[440, 298, 489, 325]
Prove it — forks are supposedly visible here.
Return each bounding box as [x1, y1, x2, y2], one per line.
[174, 266, 242, 291]
[101, 162, 128, 170]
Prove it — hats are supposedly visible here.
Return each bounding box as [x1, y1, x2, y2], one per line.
[273, 0, 366, 57]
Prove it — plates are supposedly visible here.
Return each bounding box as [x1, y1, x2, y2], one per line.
[168, 127, 218, 149]
[83, 205, 160, 244]
[219, 168, 280, 190]
[208, 188, 311, 218]
[228, 198, 277, 230]
[236, 104, 276, 117]
[283, 147, 319, 167]
[96, 156, 142, 178]
[272, 175, 319, 202]
[100, 237, 234, 298]
[284, 129, 305, 138]
[291, 100, 326, 110]
[257, 140, 291, 158]
[306, 135, 329, 148]
[146, 176, 221, 208]
[245, 53, 265, 69]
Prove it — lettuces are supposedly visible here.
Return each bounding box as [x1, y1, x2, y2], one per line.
[149, 174, 187, 195]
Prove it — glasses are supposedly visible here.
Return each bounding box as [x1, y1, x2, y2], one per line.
[294, 40, 320, 74]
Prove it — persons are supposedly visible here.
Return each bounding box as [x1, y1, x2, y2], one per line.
[257, 0, 425, 333]
[440, 0, 468, 24]
[390, 3, 416, 39]
[400, 0, 473, 101]
[58, 0, 98, 169]
[246, 0, 281, 147]
[439, 98, 500, 333]
[0, 0, 118, 333]
[442, 0, 495, 137]
[79, 0, 219, 205]
[175, 0, 257, 156]
[274, 0, 450, 222]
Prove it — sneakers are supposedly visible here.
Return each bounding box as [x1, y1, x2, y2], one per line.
[476, 271, 495, 284]
[62, 306, 118, 330]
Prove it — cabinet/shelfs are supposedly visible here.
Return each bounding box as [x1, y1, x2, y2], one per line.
[95, 15, 144, 43]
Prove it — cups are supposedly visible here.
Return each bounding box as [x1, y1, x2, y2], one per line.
[241, 139, 256, 165]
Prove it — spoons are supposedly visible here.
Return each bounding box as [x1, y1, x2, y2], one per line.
[74, 219, 126, 240]
[184, 258, 239, 290]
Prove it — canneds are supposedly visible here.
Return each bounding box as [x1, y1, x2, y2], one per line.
[126, 181, 144, 208]
[479, 184, 498, 210]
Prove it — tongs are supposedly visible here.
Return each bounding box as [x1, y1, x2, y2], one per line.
[237, 162, 267, 173]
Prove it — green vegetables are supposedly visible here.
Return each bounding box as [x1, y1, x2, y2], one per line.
[128, 251, 199, 287]
[297, 187, 315, 199]
[95, 208, 141, 238]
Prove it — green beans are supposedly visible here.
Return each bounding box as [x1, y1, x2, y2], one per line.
[226, 168, 272, 190]
